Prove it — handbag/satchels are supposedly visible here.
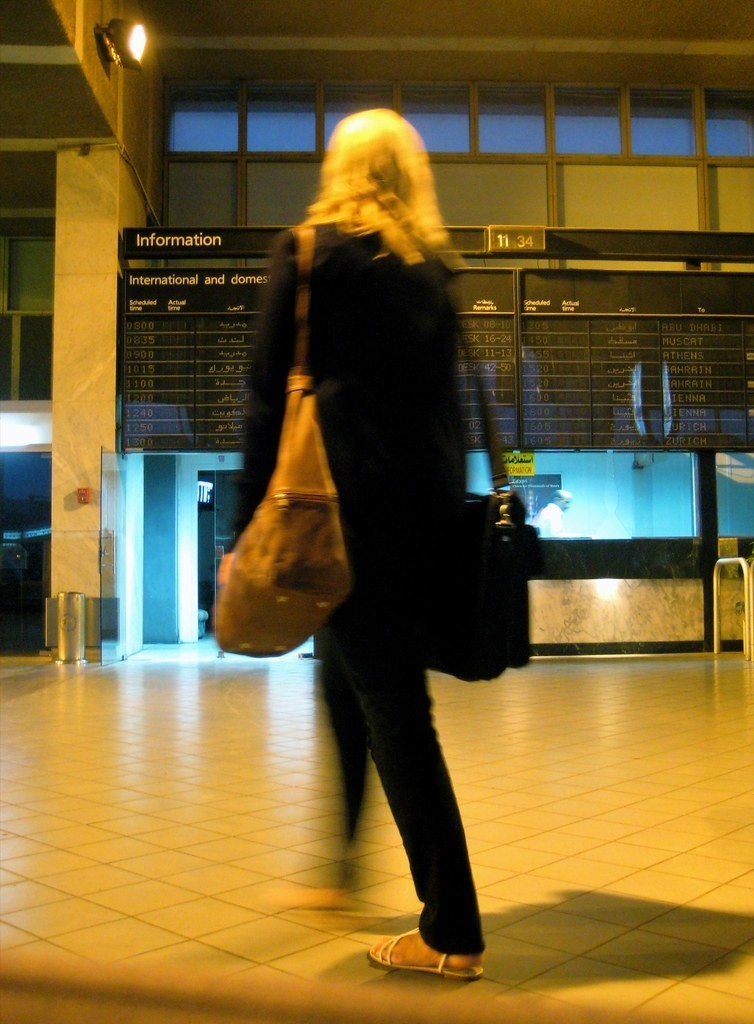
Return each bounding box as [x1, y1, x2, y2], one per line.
[429, 491, 535, 681]
[213, 394, 350, 658]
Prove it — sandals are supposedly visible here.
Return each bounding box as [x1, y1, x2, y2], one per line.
[367, 927, 484, 980]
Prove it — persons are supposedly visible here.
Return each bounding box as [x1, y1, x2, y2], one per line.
[235, 108, 485, 975]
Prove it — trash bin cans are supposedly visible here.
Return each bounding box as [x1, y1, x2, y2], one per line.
[54, 592, 88, 665]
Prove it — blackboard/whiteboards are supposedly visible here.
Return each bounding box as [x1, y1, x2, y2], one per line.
[116, 310, 754, 452]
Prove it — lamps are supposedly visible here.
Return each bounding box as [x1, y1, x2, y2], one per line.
[95, 18, 147, 71]
